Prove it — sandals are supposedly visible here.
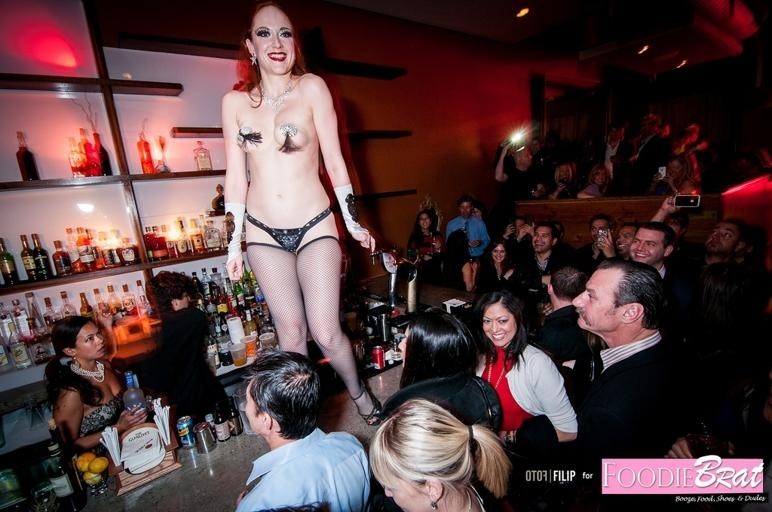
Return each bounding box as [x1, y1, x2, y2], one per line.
[351, 377, 382, 427]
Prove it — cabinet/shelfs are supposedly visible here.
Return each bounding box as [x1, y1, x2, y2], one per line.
[0, 1, 422, 367]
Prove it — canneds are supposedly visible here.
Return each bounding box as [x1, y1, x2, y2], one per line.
[372, 346, 385, 370]
[176, 416, 196, 449]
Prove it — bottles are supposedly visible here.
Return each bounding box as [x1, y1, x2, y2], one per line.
[380, 314, 390, 342]
[191, 141, 212, 171]
[206, 337, 222, 368]
[212, 184, 226, 211]
[137, 134, 154, 175]
[211, 386, 259, 443]
[46, 417, 89, 512]
[155, 160, 171, 174]
[143, 213, 246, 263]
[66, 127, 111, 177]
[179, 262, 276, 336]
[0, 225, 140, 286]
[14, 130, 42, 180]
[0, 280, 147, 373]
[121, 370, 149, 424]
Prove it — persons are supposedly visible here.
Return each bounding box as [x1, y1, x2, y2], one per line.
[42, 266, 230, 461]
[221, 2, 383, 426]
[366, 109, 772, 510]
[234, 350, 373, 512]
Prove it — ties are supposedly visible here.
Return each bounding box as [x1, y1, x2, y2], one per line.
[465, 222, 467, 232]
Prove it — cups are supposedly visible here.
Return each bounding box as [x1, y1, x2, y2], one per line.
[216, 333, 275, 367]
[81, 475, 110, 496]
[344, 311, 358, 333]
[391, 339, 402, 361]
[194, 422, 218, 455]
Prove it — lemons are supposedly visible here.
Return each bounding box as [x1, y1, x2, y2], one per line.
[89, 456, 109, 473]
[75, 452, 97, 473]
[82, 472, 102, 486]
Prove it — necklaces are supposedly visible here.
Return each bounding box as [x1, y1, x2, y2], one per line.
[259, 81, 293, 114]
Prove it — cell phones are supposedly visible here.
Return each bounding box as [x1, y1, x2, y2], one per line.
[658, 167, 666, 180]
[674, 195, 700, 207]
[597, 228, 608, 248]
